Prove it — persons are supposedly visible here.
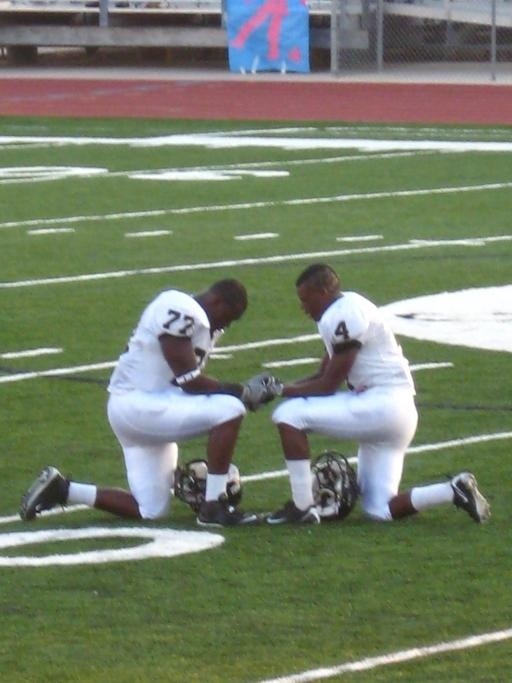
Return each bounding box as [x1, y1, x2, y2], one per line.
[19, 274, 282, 527]
[253, 261, 491, 526]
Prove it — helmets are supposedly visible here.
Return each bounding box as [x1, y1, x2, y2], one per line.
[310, 451, 356, 519]
[175, 459, 242, 514]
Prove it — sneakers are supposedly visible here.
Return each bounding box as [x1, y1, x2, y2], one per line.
[265, 500, 322, 525]
[19, 465, 69, 521]
[196, 498, 257, 525]
[451, 472, 491, 523]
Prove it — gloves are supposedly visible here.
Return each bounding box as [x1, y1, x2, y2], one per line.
[239, 373, 283, 412]
